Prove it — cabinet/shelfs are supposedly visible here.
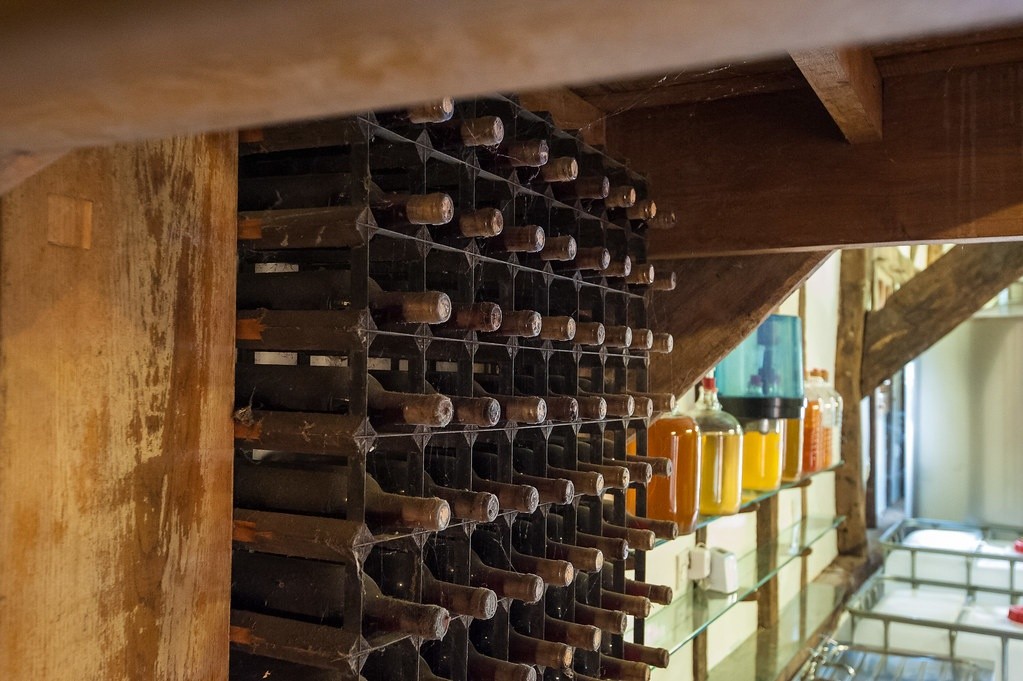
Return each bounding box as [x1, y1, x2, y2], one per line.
[232, 93, 648, 681]
[648, 461, 847, 681]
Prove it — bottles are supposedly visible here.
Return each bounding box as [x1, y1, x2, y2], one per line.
[687, 367, 842, 518]
[229, 91, 676, 680]
[626, 401, 700, 537]
